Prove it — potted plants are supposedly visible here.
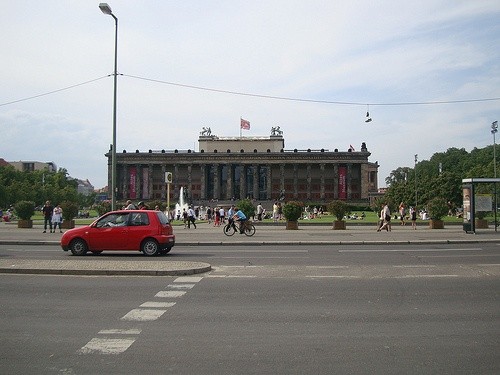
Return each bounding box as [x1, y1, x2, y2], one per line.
[281, 202, 303, 230]
[426, 196, 449, 229]
[12, 200, 37, 229]
[475, 211, 488, 229]
[236, 199, 257, 230]
[59, 201, 79, 229]
[331, 200, 353, 230]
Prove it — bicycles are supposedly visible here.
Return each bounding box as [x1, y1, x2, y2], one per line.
[223, 217, 256, 237]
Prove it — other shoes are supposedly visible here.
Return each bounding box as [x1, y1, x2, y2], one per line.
[52, 230, 55, 233]
[50, 231, 52, 233]
[43, 230, 46, 233]
[60, 231, 62, 233]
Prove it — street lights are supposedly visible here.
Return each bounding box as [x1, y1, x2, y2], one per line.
[414, 153, 418, 206]
[491, 120, 499, 233]
[98, 1, 119, 213]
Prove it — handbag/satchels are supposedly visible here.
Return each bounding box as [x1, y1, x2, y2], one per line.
[245, 223, 253, 231]
[385, 214, 391, 221]
[193, 217, 195, 221]
[60, 217, 63, 221]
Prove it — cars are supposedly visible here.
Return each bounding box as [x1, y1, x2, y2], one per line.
[61, 209, 177, 258]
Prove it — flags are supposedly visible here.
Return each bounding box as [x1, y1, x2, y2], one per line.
[241, 119, 250, 130]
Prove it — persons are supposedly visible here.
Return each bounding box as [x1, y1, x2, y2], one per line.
[173, 207, 199, 229]
[51, 205, 63, 233]
[353, 212, 366, 218]
[156, 206, 160, 211]
[410, 207, 417, 230]
[232, 207, 246, 233]
[43, 201, 53, 233]
[257, 202, 263, 221]
[0, 209, 11, 218]
[78, 211, 89, 218]
[125, 200, 149, 222]
[400, 202, 406, 225]
[377, 202, 392, 232]
[420, 210, 428, 220]
[206, 205, 226, 226]
[226, 205, 238, 232]
[108, 215, 128, 227]
[273, 202, 282, 220]
[309, 206, 323, 219]
